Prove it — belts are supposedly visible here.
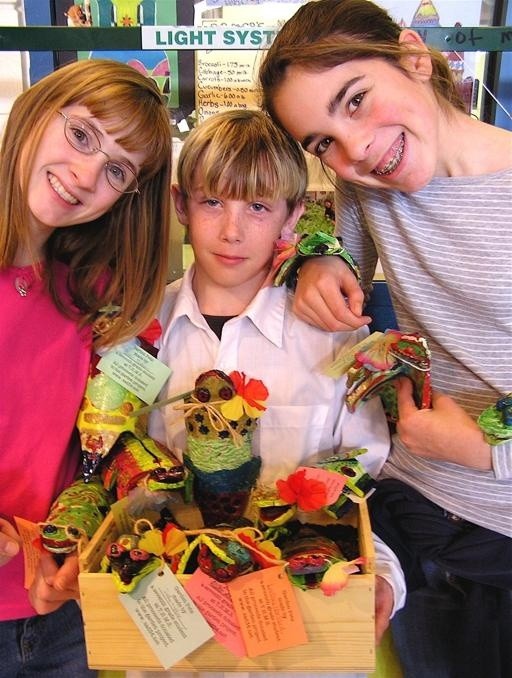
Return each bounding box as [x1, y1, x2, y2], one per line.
[386, 500, 468, 526]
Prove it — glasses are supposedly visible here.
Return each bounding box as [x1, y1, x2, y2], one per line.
[55, 108, 141, 196]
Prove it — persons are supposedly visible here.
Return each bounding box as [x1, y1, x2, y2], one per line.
[2, 60, 175, 678]
[257, 0, 512, 677]
[126, 107, 408, 678]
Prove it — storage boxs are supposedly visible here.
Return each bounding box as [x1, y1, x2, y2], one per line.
[76, 478, 376, 673]
